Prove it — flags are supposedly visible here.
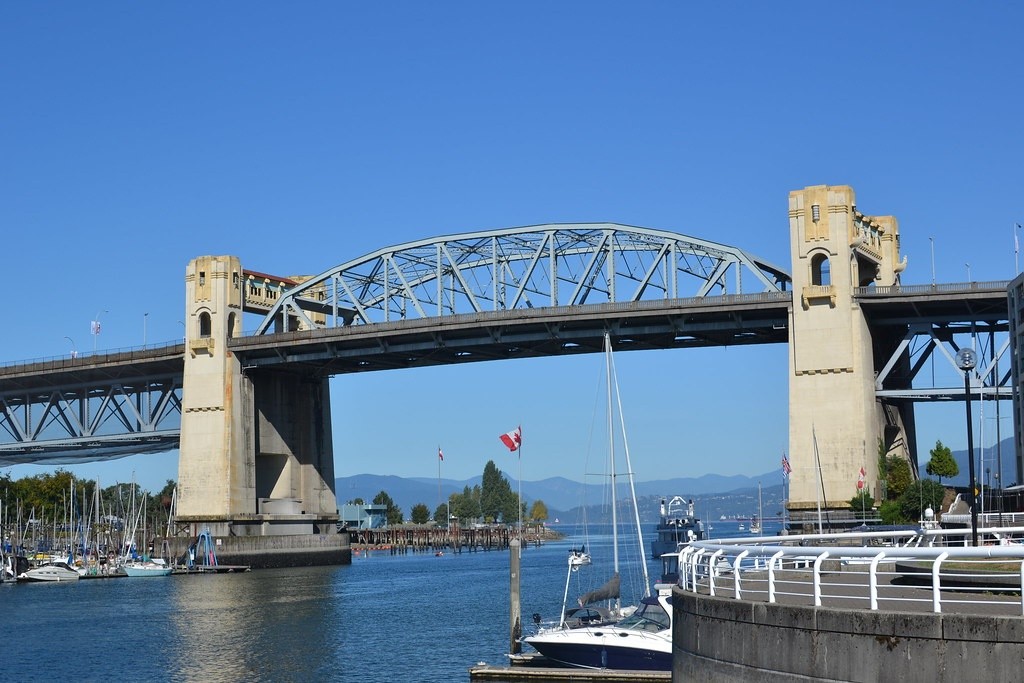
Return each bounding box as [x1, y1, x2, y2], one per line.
[499, 427, 521, 452]
[858, 467, 864, 487]
[782, 455, 794, 477]
[438, 448, 444, 461]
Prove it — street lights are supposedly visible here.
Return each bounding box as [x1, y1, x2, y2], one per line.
[954, 347, 979, 547]
[93, 310, 108, 354]
[65, 337, 74, 352]
[965, 264, 970, 282]
[929, 237, 935, 283]
[1013, 222, 1021, 276]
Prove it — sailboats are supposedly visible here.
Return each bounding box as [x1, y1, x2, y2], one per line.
[521, 330, 674, 670]
[0, 470, 178, 581]
[567, 499, 592, 566]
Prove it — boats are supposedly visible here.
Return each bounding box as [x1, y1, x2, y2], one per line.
[738, 524, 745, 530]
[749, 514, 760, 534]
[651, 495, 707, 560]
